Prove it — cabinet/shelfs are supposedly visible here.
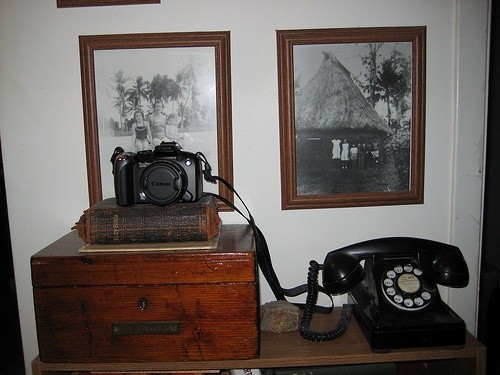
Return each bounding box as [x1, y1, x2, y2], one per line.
[28, 304, 487, 375]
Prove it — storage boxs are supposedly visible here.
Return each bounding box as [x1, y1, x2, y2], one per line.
[29, 225, 261, 366]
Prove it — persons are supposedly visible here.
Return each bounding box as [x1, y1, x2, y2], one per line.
[330, 135, 382, 172]
[131, 101, 178, 151]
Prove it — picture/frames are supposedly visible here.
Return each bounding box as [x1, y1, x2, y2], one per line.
[274, 25, 427, 210]
[79, 31, 234, 214]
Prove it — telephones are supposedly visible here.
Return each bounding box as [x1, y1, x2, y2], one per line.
[323, 236, 469, 355]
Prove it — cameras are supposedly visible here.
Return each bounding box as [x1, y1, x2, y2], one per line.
[113, 142, 203, 207]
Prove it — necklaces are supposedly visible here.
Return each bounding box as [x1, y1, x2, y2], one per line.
[134, 121, 147, 131]
